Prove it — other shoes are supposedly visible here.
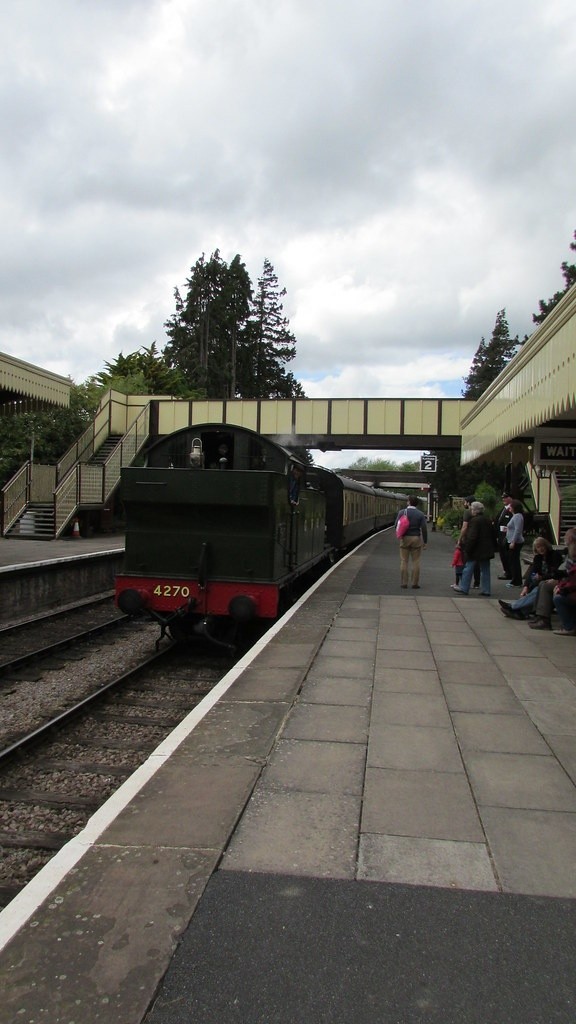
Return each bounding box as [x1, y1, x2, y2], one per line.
[451, 584, 458, 588]
[412, 584, 421, 589]
[479, 593, 490, 597]
[400, 585, 408, 588]
[505, 584, 523, 589]
[470, 585, 480, 589]
[454, 587, 468, 595]
[498, 599, 527, 620]
[497, 574, 512, 580]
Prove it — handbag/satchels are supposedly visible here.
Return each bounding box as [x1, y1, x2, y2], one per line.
[396, 509, 409, 538]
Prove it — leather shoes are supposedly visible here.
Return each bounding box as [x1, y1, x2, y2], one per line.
[528, 616, 552, 630]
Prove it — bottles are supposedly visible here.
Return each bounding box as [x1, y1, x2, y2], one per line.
[531, 573, 537, 582]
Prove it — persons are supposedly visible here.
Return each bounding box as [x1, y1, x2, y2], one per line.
[520, 537, 567, 603]
[503, 500, 525, 589]
[454, 502, 496, 596]
[458, 495, 480, 588]
[528, 530, 575, 630]
[553, 542, 576, 635]
[394, 495, 427, 588]
[451, 542, 465, 588]
[492, 491, 516, 579]
[498, 526, 576, 620]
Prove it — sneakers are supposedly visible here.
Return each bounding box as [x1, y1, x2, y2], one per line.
[553, 625, 576, 635]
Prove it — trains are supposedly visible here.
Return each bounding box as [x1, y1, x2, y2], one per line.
[112, 422, 429, 671]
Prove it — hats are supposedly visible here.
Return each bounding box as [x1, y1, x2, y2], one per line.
[464, 495, 476, 503]
[501, 491, 514, 498]
